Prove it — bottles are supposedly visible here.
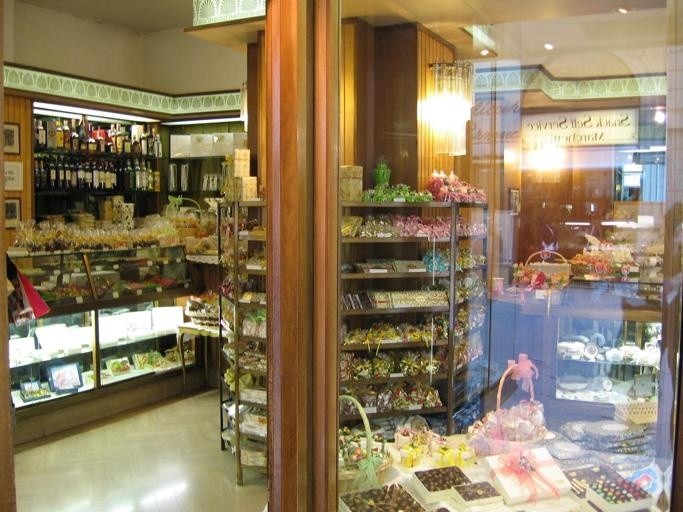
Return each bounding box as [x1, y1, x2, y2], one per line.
[34, 115, 162, 191]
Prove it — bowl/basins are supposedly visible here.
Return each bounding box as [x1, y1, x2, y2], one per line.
[559, 375, 589, 391]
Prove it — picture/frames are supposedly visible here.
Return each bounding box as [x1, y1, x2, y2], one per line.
[3, 121, 20, 155]
[4, 161, 23, 191]
[4, 197, 21, 229]
[508, 187, 521, 216]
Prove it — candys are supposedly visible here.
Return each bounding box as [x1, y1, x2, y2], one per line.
[341, 467, 498, 512]
[568, 463, 648, 504]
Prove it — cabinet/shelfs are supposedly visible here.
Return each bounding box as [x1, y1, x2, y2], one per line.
[490, 288, 664, 433]
[5, 88, 244, 221]
[33, 148, 161, 218]
[216, 201, 489, 451]
[7, 251, 196, 448]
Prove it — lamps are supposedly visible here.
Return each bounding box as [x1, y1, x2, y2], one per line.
[430, 59, 475, 155]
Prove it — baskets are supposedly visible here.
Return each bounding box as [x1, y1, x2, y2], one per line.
[524, 251, 571, 282]
[482, 364, 547, 454]
[339, 394, 394, 493]
[161, 198, 202, 242]
[614, 402, 658, 424]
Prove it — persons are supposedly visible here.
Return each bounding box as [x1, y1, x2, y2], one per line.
[618, 202, 682, 403]
